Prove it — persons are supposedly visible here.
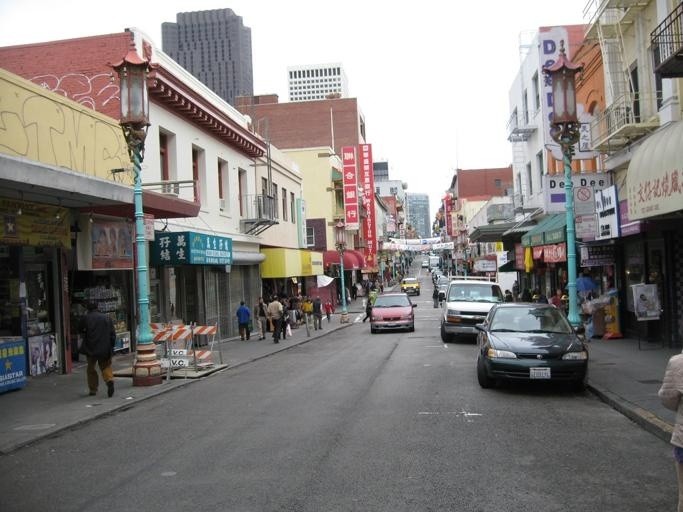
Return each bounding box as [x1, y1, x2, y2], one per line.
[432, 286, 439, 308]
[657, 319, 683, 511]
[75, 302, 116, 397]
[236, 278, 384, 344]
[504, 287, 583, 317]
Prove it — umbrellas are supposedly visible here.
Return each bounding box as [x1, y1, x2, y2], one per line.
[564, 276, 599, 295]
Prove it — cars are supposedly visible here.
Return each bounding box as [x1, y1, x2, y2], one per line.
[474, 301, 589, 387]
[399, 277, 421, 295]
[370, 292, 418, 334]
[421, 250, 450, 300]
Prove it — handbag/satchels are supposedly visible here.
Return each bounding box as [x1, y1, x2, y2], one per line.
[286, 324, 293, 338]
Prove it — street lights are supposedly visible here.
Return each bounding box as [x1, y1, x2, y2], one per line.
[111, 32, 165, 388]
[542, 38, 583, 338]
[458, 224, 469, 280]
[377, 239, 385, 293]
[335, 215, 350, 324]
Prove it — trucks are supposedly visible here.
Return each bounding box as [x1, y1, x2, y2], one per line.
[441, 276, 503, 342]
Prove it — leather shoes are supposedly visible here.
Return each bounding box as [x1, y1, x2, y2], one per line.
[240, 335, 280, 342]
[88, 391, 96, 396]
[107, 379, 114, 398]
